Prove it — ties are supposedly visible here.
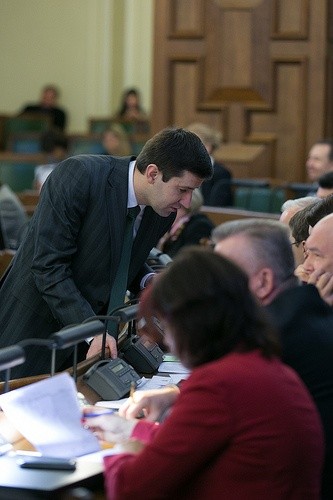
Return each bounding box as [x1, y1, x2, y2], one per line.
[105, 205, 140, 340]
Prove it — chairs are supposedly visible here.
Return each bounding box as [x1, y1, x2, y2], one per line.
[234, 187, 286, 216]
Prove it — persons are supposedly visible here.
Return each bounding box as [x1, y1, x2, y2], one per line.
[306, 141, 333, 182]
[18, 86, 68, 159]
[279, 197, 322, 267]
[119, 218, 333, 500]
[288, 200, 321, 268]
[317, 171, 333, 196]
[117, 90, 149, 123]
[0, 127, 213, 383]
[102, 124, 131, 156]
[294, 213, 333, 305]
[305, 192, 333, 236]
[185, 123, 234, 208]
[177, 189, 216, 247]
[80, 250, 324, 500]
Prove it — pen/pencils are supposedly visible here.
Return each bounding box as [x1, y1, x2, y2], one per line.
[83, 408, 115, 418]
[129, 382, 138, 410]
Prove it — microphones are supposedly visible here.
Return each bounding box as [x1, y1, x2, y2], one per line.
[74, 315, 121, 383]
[101, 304, 130, 360]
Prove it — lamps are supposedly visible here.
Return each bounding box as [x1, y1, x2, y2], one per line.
[208, 180, 269, 207]
[0, 303, 142, 393]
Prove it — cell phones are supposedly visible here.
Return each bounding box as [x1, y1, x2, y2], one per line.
[19, 460, 77, 472]
